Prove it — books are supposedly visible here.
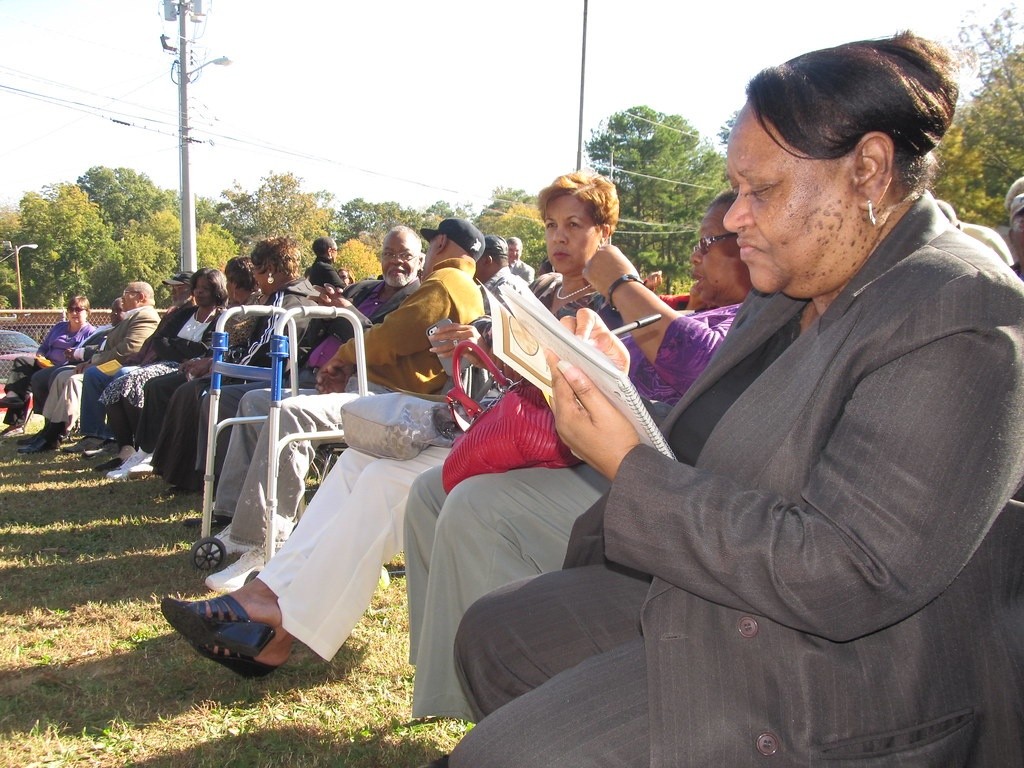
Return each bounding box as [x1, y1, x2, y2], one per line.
[498, 276, 677, 460]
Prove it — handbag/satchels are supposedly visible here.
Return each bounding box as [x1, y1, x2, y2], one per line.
[443, 341, 580, 496]
[341, 392, 459, 461]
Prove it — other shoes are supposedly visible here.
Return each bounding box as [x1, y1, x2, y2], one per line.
[0, 395, 24, 408]
[62, 436, 106, 454]
[84, 439, 119, 459]
[0, 425, 24, 438]
[17, 430, 44, 445]
[162, 485, 194, 497]
[18, 436, 53, 453]
[95, 456, 123, 471]
[184, 513, 232, 528]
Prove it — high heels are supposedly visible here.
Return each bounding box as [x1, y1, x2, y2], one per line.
[189, 638, 278, 680]
[161, 593, 276, 658]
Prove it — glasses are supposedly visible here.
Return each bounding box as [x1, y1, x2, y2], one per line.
[67, 306, 87, 313]
[380, 250, 418, 261]
[694, 232, 739, 254]
[123, 290, 147, 297]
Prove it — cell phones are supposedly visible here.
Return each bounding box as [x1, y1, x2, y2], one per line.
[426, 319, 472, 377]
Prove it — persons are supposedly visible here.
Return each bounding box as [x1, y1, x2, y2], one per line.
[184, 225, 422, 524]
[17, 278, 161, 453]
[1004, 176, 1024, 282]
[337, 267, 355, 286]
[936, 200, 1014, 267]
[0, 295, 107, 437]
[191, 217, 485, 592]
[304, 236, 348, 288]
[403, 189, 754, 768]
[17, 297, 127, 444]
[60, 266, 229, 471]
[106, 235, 319, 497]
[305, 266, 311, 281]
[474, 235, 530, 315]
[447, 26, 1024, 768]
[507, 236, 534, 284]
[160, 169, 619, 678]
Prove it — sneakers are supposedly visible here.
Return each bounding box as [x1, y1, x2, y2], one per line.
[106, 453, 153, 479]
[204, 546, 278, 595]
[197, 522, 253, 557]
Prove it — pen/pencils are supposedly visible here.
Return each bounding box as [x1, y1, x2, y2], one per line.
[610, 313, 662, 335]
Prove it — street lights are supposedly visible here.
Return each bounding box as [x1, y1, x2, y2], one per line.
[180, 56, 233, 273]
[15, 244, 38, 309]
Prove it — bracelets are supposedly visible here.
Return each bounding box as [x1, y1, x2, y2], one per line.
[607, 273, 644, 312]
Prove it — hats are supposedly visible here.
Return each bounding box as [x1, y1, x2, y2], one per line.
[420, 219, 486, 262]
[485, 235, 509, 255]
[162, 272, 196, 286]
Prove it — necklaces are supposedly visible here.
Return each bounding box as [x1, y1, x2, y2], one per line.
[187, 307, 215, 346]
[557, 283, 591, 299]
